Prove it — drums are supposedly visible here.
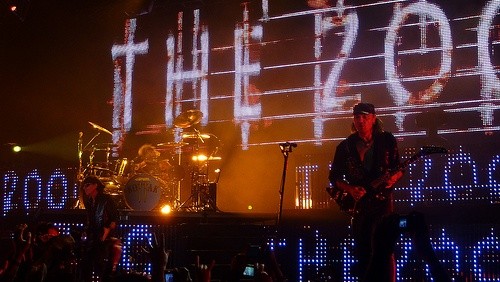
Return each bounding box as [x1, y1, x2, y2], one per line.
[111, 156, 134, 176]
[92, 149, 114, 164]
[123, 172, 170, 214]
[80, 175, 122, 211]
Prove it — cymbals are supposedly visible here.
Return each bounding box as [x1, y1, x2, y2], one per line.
[172, 109, 204, 128]
[89, 121, 115, 136]
[157, 141, 188, 147]
[181, 134, 211, 138]
[91, 143, 117, 146]
[191, 155, 221, 160]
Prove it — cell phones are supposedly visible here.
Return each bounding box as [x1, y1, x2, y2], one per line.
[164, 269, 174, 282]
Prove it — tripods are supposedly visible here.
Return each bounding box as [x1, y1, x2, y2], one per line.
[175, 118, 217, 212]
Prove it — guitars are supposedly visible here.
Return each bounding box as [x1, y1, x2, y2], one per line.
[325, 144, 449, 216]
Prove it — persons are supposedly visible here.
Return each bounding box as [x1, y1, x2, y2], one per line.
[81, 175, 120, 241]
[330, 103, 402, 282]
[0, 222, 273, 282]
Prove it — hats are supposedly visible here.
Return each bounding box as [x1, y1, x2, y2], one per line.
[84, 177, 105, 189]
[353, 103, 375, 114]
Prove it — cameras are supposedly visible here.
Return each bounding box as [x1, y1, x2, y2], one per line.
[242, 265, 255, 278]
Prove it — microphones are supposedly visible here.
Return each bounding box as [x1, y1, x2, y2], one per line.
[279, 143, 297, 147]
[79, 132, 83, 138]
